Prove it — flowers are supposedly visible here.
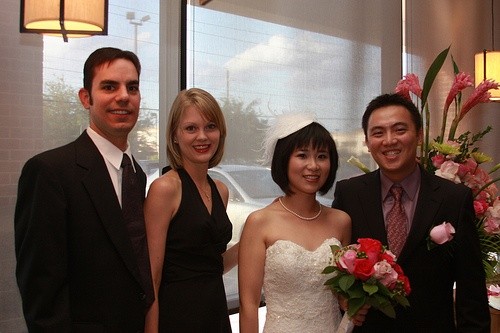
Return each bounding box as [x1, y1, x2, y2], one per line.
[325, 45, 500, 317]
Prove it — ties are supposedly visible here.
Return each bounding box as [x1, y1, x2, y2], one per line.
[121, 151, 156, 308]
[385, 185, 409, 261]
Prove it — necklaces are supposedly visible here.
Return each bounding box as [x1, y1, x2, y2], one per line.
[278, 198, 322, 220]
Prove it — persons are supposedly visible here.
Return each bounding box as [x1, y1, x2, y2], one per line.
[238, 113, 371, 333]
[14, 47, 155, 333]
[331, 94, 491, 333]
[143, 88, 241, 333]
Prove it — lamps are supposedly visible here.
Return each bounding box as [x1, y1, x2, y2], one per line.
[475, 49, 500, 101]
[19, 0, 108, 42]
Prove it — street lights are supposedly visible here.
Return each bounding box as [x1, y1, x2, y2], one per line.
[126, 12, 151, 54]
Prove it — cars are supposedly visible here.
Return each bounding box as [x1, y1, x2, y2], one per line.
[145, 164, 333, 311]
